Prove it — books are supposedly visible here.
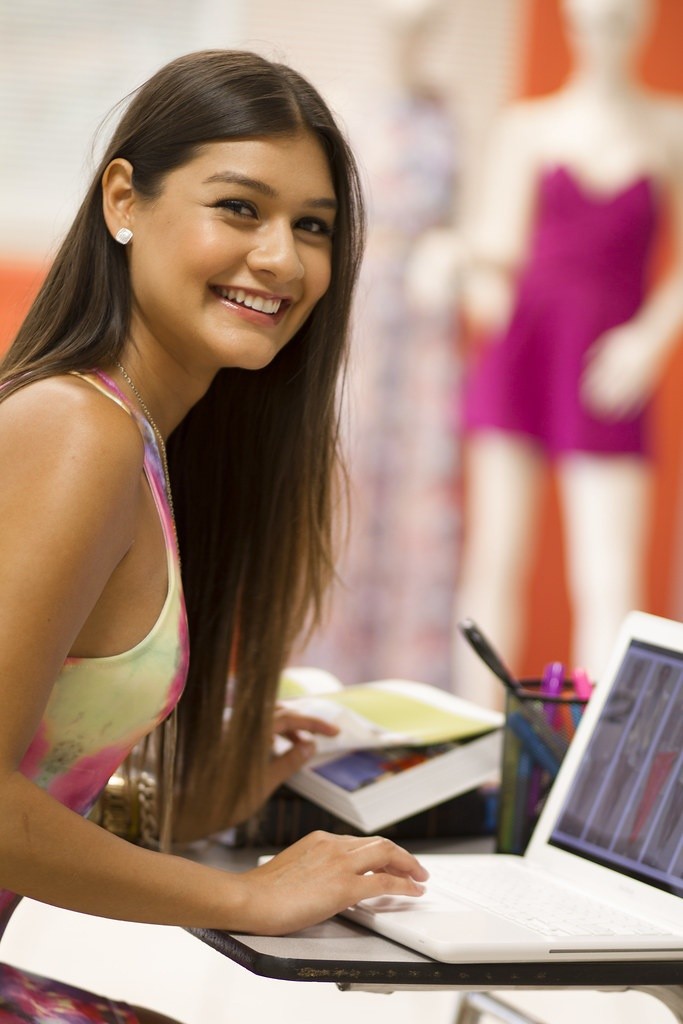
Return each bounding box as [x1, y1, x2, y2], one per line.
[183, 646, 514, 837]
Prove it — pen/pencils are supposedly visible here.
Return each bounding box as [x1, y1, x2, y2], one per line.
[455, 617, 594, 856]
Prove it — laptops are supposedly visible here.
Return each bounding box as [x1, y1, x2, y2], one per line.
[257, 611, 683, 964]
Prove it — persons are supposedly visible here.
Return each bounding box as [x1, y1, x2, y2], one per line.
[592, 662, 671, 843]
[560, 656, 645, 833]
[677, 862, 682, 879]
[647, 762, 681, 871]
[624, 682, 682, 860]
[410, 0, 683, 844]
[255, 0, 470, 730]
[0, 48, 429, 1014]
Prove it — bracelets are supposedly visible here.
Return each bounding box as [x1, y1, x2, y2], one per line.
[135, 778, 169, 852]
[101, 779, 133, 848]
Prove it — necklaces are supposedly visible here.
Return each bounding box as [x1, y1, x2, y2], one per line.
[112, 357, 196, 571]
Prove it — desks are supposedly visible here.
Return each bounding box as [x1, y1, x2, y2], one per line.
[95, 835, 683, 1024]
